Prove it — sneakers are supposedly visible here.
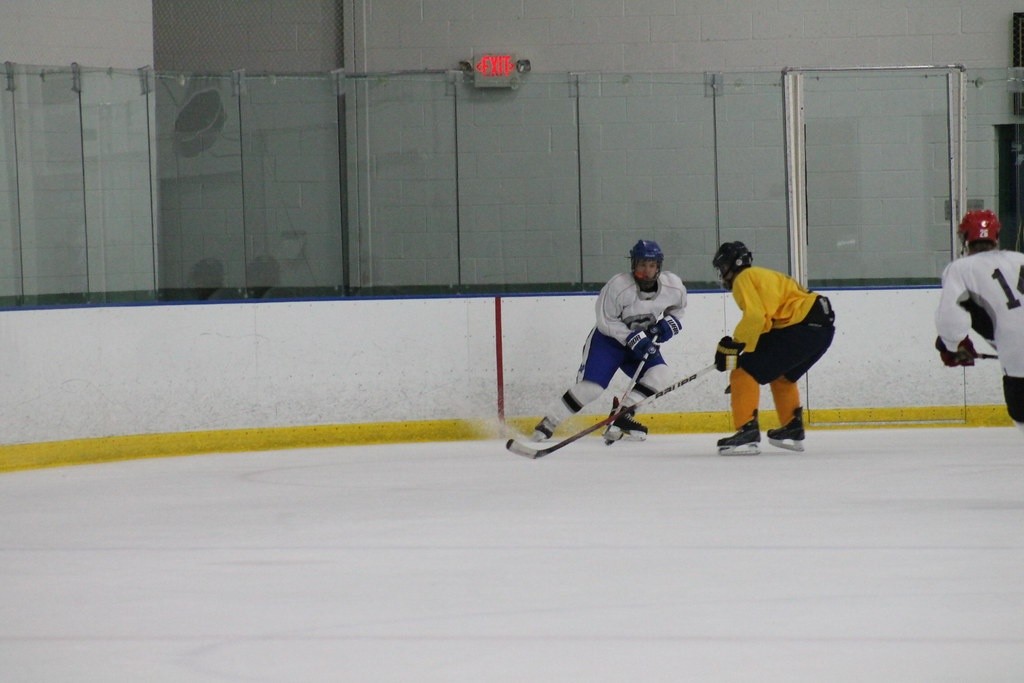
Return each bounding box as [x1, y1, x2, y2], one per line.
[767, 406, 805, 451]
[607, 396, 648, 441]
[531, 416, 557, 442]
[716, 409, 762, 455]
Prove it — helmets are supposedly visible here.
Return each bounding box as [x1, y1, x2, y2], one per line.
[712, 241, 753, 272]
[630, 239, 664, 270]
[958, 210, 1001, 246]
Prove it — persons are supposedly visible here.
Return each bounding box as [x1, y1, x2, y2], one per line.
[935, 209, 1024, 429]
[713, 241, 835, 455]
[532, 239, 687, 442]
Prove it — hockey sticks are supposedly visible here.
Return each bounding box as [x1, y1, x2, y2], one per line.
[505, 363, 717, 461]
[973, 353, 998, 359]
[602, 334, 659, 442]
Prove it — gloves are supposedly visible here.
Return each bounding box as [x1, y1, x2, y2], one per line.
[714, 336, 746, 372]
[935, 334, 978, 367]
[625, 329, 660, 368]
[644, 314, 682, 343]
